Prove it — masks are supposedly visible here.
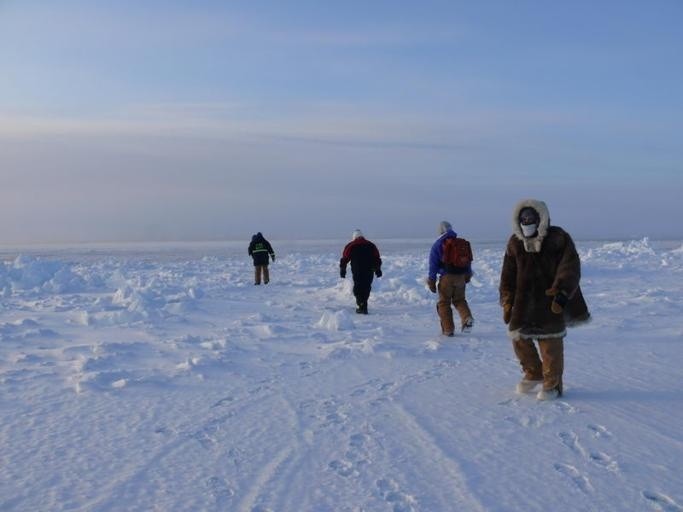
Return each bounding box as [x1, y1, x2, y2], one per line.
[519, 224, 536, 237]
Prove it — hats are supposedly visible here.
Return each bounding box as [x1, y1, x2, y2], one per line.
[352, 229, 363, 239]
[437, 222, 451, 234]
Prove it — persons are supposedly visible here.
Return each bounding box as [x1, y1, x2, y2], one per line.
[248, 232, 275, 285]
[497, 199, 592, 402]
[427, 221, 475, 337]
[340, 229, 382, 314]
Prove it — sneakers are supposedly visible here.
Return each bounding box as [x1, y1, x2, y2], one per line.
[537, 385, 559, 400]
[516, 375, 544, 393]
[461, 317, 473, 333]
[442, 331, 453, 336]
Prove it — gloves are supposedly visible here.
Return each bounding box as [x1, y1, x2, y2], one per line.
[427, 278, 436, 293]
[464, 272, 470, 283]
[502, 304, 512, 323]
[375, 269, 381, 278]
[270, 254, 274, 262]
[545, 286, 570, 313]
[340, 268, 346, 278]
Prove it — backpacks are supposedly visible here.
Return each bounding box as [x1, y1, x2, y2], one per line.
[441, 237, 472, 269]
